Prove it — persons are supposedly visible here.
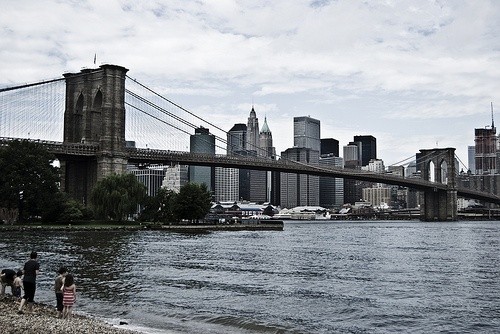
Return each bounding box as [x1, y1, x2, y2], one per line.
[55, 268, 69, 318]
[60, 274, 76, 319]
[18, 252, 40, 314]
[0, 269, 17, 295]
[13, 270, 24, 297]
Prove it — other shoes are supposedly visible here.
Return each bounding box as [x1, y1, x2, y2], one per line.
[18, 308, 24, 314]
